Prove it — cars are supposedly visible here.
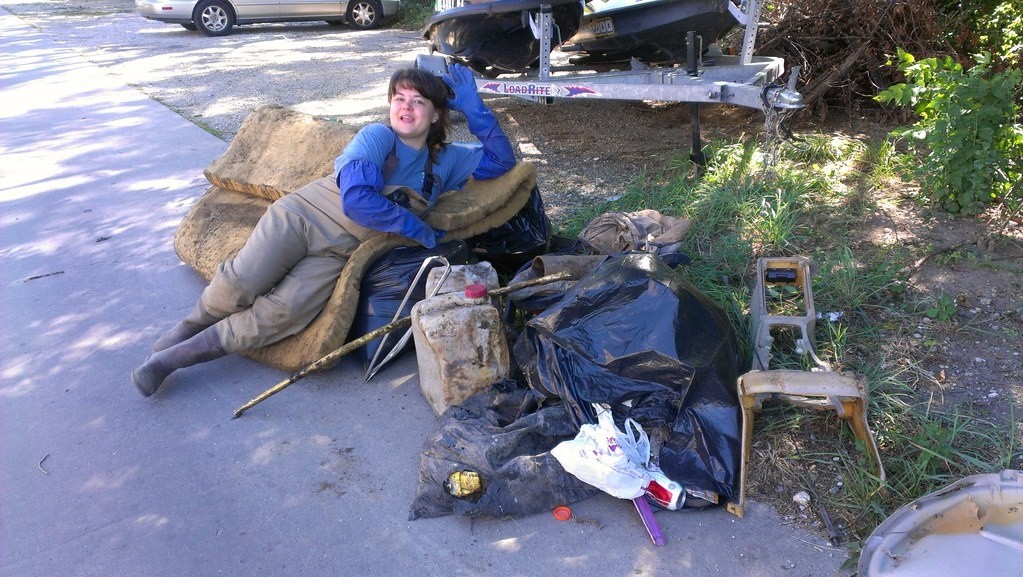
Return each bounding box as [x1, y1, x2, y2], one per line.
[134, 0, 402, 37]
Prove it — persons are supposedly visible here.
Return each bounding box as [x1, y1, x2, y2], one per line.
[130, 62, 517, 396]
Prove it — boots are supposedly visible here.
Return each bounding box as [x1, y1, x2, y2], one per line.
[150, 295, 222, 354]
[131, 323, 228, 397]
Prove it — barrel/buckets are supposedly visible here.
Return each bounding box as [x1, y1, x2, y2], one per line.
[411, 284, 510, 418]
[426, 261, 500, 306]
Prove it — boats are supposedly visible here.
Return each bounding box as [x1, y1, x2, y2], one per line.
[556, 0, 744, 65]
[417, 0, 584, 80]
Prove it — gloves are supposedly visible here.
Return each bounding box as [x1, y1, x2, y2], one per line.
[338, 160, 446, 249]
[442, 63, 516, 181]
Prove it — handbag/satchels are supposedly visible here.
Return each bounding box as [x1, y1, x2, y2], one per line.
[550, 401, 651, 500]
